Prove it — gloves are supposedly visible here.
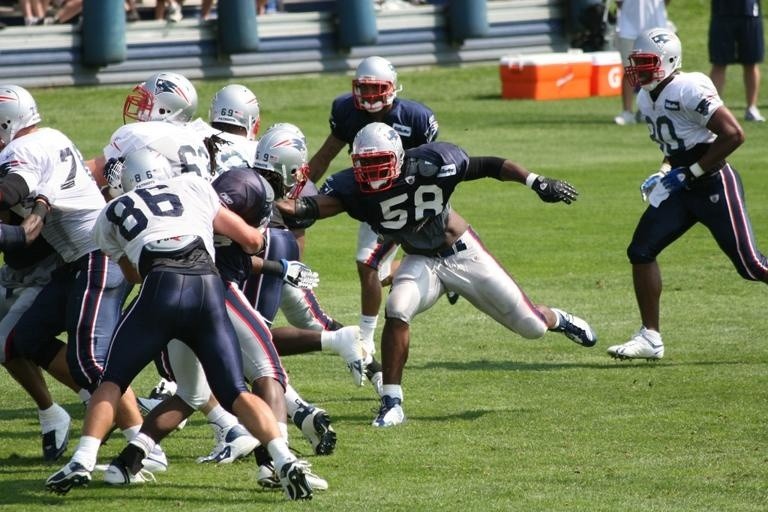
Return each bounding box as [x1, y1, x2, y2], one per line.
[103, 157, 125, 189]
[277, 259, 320, 290]
[641, 163, 705, 207]
[524, 172, 578, 204]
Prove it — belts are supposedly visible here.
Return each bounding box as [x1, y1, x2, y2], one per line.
[409, 242, 467, 258]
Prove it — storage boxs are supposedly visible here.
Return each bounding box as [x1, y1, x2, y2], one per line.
[498, 52, 591, 100]
[585, 49, 621, 96]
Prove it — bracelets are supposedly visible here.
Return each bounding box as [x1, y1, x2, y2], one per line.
[689, 162, 705, 178]
[659, 163, 672, 175]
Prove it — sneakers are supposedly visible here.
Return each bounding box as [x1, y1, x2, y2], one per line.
[292, 407, 336, 454]
[447, 291, 458, 304]
[607, 326, 665, 359]
[278, 464, 313, 500]
[258, 462, 329, 490]
[41, 378, 188, 496]
[196, 422, 260, 465]
[614, 112, 637, 125]
[548, 307, 597, 347]
[339, 325, 404, 427]
[745, 107, 765, 122]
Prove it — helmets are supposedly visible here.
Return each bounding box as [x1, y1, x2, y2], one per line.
[625, 29, 682, 91]
[0, 84, 42, 145]
[123, 72, 198, 125]
[121, 150, 172, 191]
[353, 56, 397, 113]
[254, 122, 310, 198]
[210, 85, 260, 139]
[352, 123, 405, 193]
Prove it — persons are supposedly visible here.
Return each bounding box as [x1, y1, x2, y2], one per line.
[612, 0, 670, 126]
[707, 0, 765, 123]
[606, 27, 768, 361]
